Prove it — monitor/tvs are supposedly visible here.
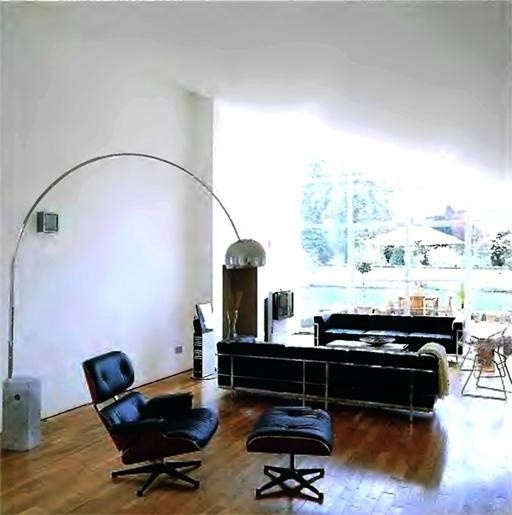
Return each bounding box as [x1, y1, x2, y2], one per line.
[273, 291, 293, 320]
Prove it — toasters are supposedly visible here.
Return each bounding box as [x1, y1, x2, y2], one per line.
[471, 313, 486, 322]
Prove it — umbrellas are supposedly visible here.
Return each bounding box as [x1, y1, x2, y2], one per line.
[372, 225, 464, 249]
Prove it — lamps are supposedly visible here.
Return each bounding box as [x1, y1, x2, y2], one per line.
[0, 153, 266, 453]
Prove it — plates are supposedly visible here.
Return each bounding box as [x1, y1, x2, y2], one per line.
[359, 336, 396, 347]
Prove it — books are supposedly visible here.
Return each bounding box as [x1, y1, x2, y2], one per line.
[272, 290, 294, 320]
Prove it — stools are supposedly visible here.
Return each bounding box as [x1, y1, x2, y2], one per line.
[247, 406, 334, 499]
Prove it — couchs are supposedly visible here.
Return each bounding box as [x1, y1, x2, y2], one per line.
[217, 335, 439, 420]
[314, 314, 465, 363]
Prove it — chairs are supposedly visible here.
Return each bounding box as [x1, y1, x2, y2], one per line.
[462, 336, 512, 400]
[83, 351, 219, 496]
[459, 326, 507, 378]
